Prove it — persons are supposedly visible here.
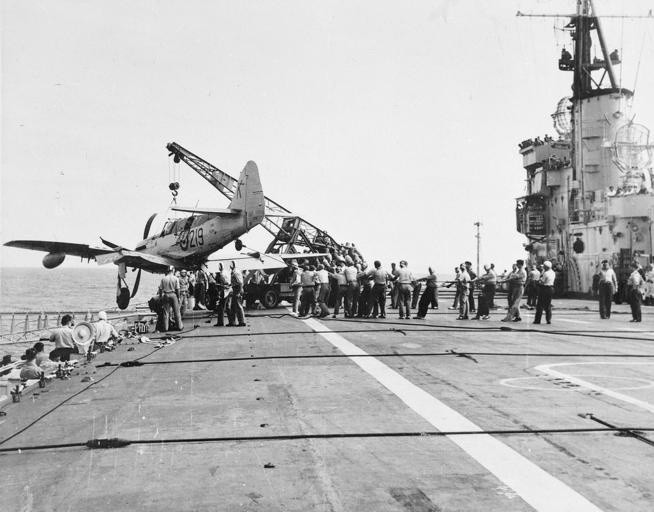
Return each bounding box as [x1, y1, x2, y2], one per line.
[289, 231, 418, 320]
[597, 260, 618, 319]
[446, 258, 556, 324]
[156, 261, 246, 333]
[50, 310, 118, 354]
[1, 342, 50, 379]
[250, 274, 257, 284]
[626, 263, 644, 322]
[425, 265, 439, 309]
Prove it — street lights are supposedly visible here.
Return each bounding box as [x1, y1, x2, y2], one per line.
[472, 222, 483, 279]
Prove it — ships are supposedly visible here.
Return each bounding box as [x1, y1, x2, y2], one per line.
[0, 2, 654, 511]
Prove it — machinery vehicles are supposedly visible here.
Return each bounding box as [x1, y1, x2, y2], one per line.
[164, 141, 375, 311]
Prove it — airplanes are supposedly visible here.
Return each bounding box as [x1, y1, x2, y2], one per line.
[2, 159, 264, 309]
